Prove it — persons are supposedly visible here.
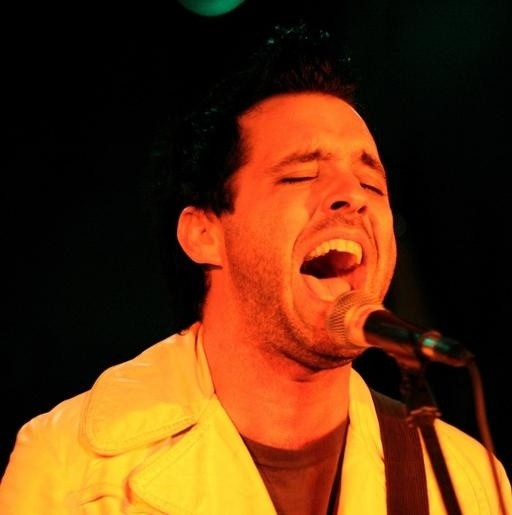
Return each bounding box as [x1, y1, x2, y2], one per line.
[0, 54, 512, 515]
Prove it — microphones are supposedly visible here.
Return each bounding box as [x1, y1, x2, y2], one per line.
[325, 289, 479, 371]
